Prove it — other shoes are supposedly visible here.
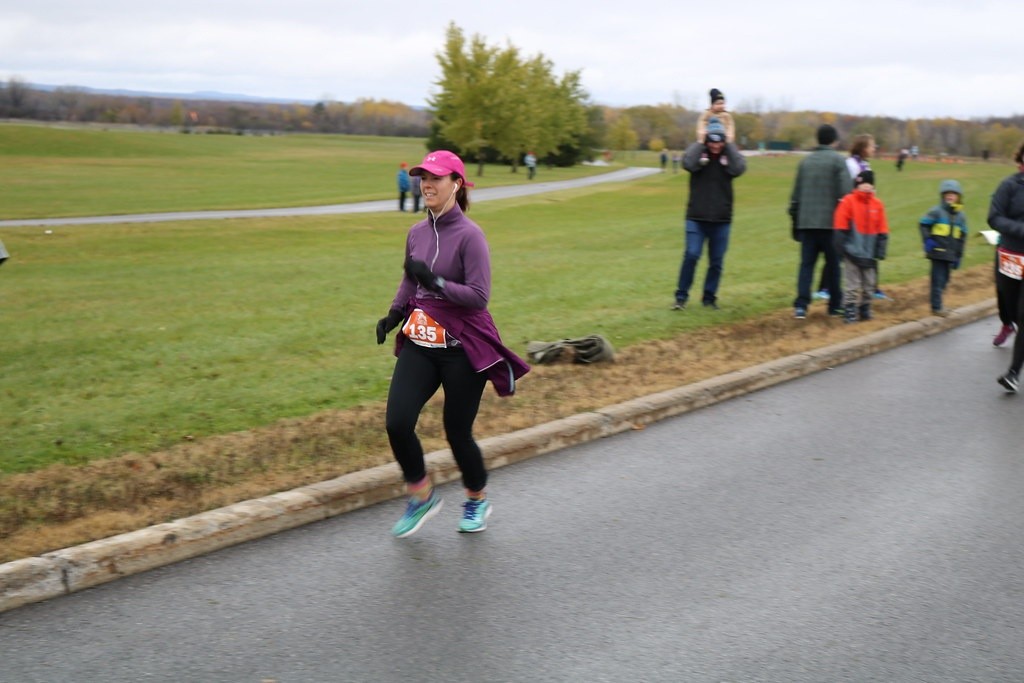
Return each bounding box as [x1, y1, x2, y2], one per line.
[699, 152, 709, 165]
[720, 155, 729, 166]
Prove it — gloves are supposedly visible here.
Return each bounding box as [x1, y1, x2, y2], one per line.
[874, 251, 885, 260]
[790, 217, 802, 240]
[953, 259, 959, 269]
[925, 238, 937, 253]
[404, 258, 443, 292]
[834, 244, 845, 256]
[376, 309, 403, 344]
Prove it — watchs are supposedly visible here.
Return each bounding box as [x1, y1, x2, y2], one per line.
[435, 276, 445, 289]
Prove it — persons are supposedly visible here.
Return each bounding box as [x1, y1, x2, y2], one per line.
[660, 147, 668, 168]
[894, 154, 907, 171]
[397, 163, 411, 212]
[987, 142, 1023, 393]
[695, 86, 735, 166]
[410, 176, 422, 212]
[671, 115, 748, 311]
[374, 150, 531, 540]
[831, 170, 889, 324]
[918, 177, 969, 318]
[788, 126, 853, 319]
[815, 133, 890, 301]
[524, 152, 536, 178]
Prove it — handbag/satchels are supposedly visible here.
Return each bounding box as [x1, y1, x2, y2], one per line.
[997, 248, 1024, 280]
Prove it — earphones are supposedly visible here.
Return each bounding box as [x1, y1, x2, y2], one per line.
[452, 183, 458, 193]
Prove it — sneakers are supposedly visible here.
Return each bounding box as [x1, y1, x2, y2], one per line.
[998, 373, 1018, 392]
[392, 487, 444, 539]
[993, 323, 1016, 347]
[458, 493, 491, 532]
[672, 287, 948, 323]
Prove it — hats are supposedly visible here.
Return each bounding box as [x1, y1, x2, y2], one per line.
[704, 117, 725, 142]
[410, 150, 474, 187]
[815, 124, 837, 144]
[855, 170, 873, 188]
[710, 89, 724, 103]
